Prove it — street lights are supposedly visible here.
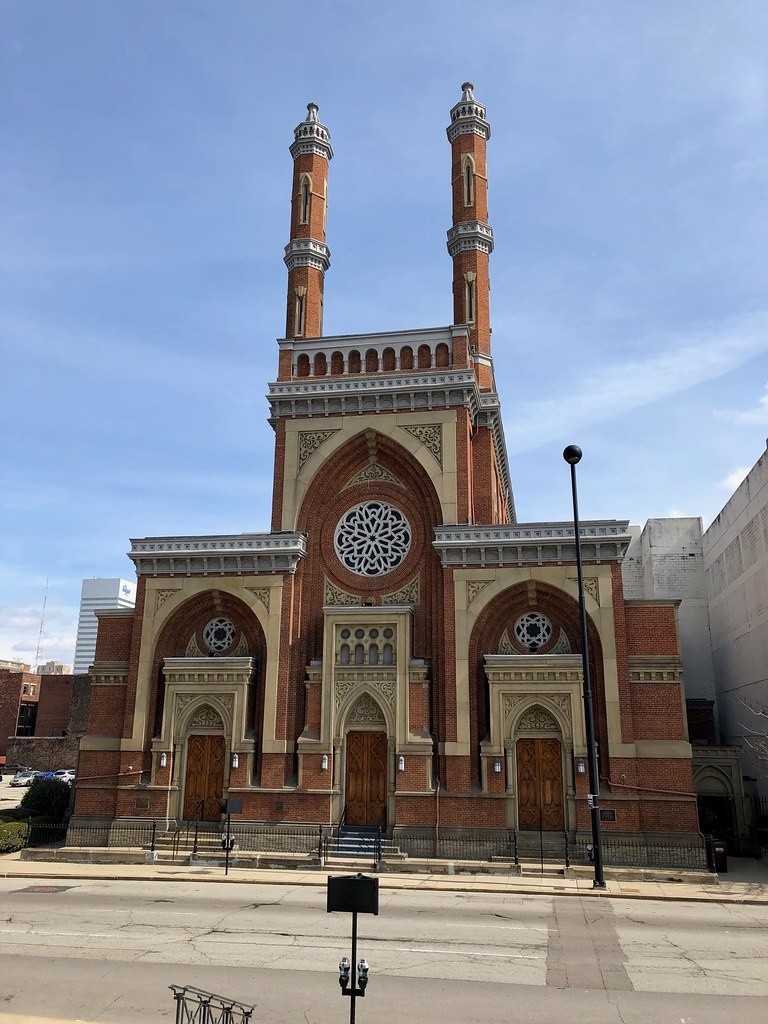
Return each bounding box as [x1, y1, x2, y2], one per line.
[561, 443, 608, 890]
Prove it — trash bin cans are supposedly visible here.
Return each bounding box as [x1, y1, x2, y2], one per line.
[708, 840, 729, 875]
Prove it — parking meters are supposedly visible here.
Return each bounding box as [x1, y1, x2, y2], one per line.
[219, 797, 244, 875]
[325, 871, 380, 1024]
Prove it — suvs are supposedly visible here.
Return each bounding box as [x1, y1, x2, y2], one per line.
[53, 769, 76, 788]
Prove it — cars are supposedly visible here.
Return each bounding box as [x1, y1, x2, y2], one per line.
[10, 770, 42, 787]
[36, 772, 53, 782]
[0, 764, 32, 775]
